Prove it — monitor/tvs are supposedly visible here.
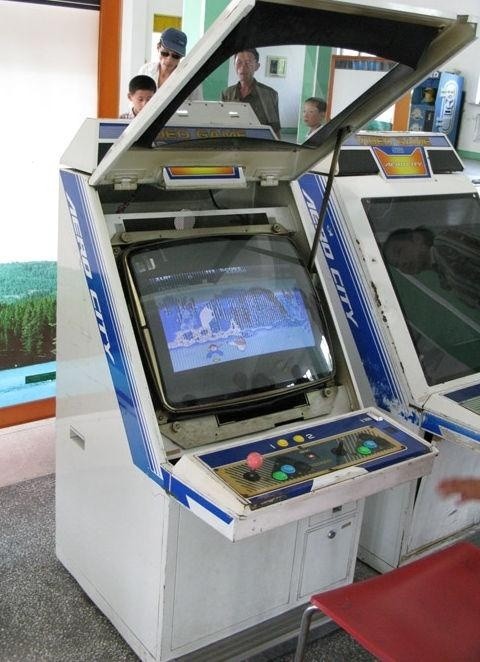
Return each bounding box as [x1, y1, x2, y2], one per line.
[116, 230, 336, 418]
[362, 189, 479, 388]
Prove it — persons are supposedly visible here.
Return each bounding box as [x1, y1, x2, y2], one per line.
[117, 75, 156, 120]
[302, 98, 327, 143]
[129, 25, 204, 101]
[382, 220, 479, 318]
[219, 48, 280, 140]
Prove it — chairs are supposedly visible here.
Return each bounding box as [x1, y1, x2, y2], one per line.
[294, 541, 480, 662]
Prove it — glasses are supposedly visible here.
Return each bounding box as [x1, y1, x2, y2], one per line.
[160, 51, 182, 59]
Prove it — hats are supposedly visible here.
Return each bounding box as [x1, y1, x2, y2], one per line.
[159, 27, 187, 57]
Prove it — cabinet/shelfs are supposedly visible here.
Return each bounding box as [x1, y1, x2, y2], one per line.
[408, 71, 464, 147]
